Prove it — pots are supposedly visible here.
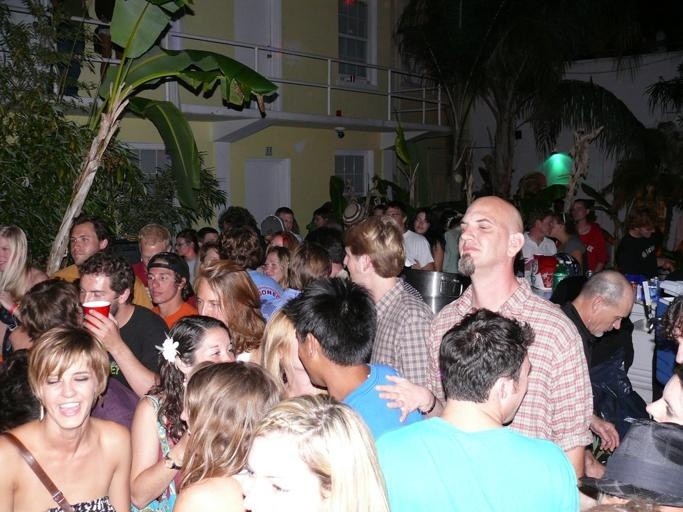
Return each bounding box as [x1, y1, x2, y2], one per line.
[405, 266, 464, 318]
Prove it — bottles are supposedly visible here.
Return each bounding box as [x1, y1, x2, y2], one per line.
[552, 259, 568, 292]
[648, 279, 656, 298]
[654, 277, 660, 298]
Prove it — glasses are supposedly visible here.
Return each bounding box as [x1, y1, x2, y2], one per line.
[558, 212, 566, 223]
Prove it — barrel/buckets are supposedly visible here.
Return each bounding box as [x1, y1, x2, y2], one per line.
[412, 270, 464, 316]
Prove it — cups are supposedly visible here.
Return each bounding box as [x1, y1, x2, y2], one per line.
[81, 300, 111, 328]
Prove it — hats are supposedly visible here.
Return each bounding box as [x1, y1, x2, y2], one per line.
[146, 252, 194, 298]
[578, 418, 682, 509]
[260, 215, 285, 237]
[341, 202, 369, 227]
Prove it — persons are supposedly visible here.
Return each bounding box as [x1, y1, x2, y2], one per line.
[48, 0, 90, 99]
[0, 186, 683, 512]
[91, 0, 130, 90]
